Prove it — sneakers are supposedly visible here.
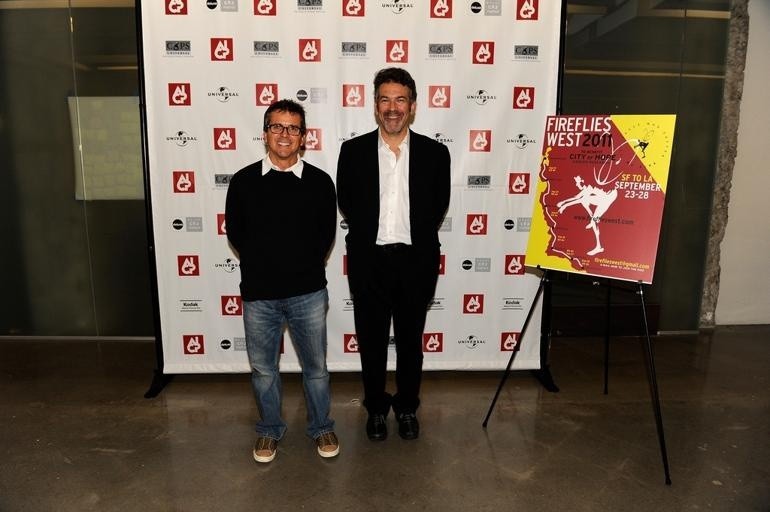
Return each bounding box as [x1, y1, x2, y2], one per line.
[396, 411, 419, 440]
[316, 431, 340, 458]
[366, 412, 387, 441]
[254, 435, 277, 463]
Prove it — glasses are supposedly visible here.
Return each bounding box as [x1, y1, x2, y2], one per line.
[267, 123, 303, 137]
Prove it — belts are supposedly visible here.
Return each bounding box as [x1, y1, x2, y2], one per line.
[382, 243, 407, 251]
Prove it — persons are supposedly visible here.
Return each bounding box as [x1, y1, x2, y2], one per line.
[334, 67, 451, 442]
[224, 97, 342, 462]
